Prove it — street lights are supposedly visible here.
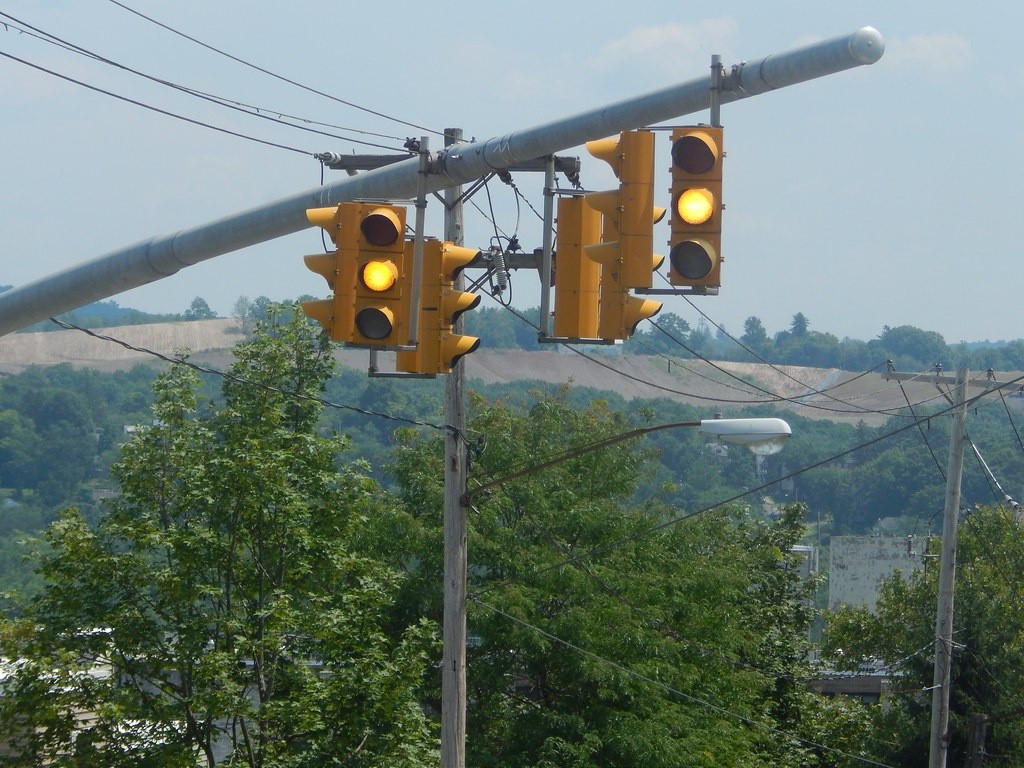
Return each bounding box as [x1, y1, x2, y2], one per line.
[459, 415, 797, 512]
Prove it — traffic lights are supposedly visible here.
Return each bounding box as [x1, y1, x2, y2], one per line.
[601, 203, 666, 345]
[586, 132, 654, 290]
[670, 120, 723, 291]
[416, 234, 484, 376]
[301, 199, 352, 345]
[352, 204, 408, 347]
